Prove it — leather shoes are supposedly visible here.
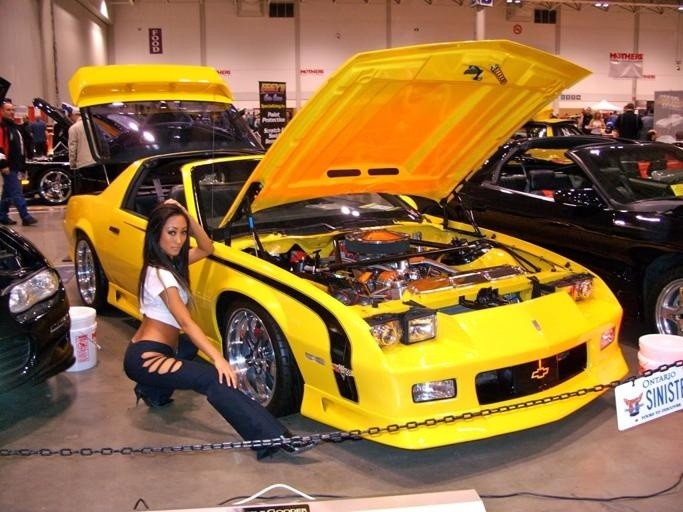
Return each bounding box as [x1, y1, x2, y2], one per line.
[1, 217, 37, 226]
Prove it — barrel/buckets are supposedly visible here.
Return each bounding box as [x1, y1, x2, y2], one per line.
[637, 333, 683, 378]
[62, 306, 101, 371]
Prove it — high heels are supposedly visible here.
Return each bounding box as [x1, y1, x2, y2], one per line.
[262, 431, 311, 461]
[132, 384, 172, 409]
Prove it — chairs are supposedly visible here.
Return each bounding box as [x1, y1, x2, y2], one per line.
[526, 168, 629, 197]
[168, 184, 220, 226]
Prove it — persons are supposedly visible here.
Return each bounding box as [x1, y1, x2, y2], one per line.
[124, 199, 313, 459]
[581, 103, 655, 141]
[0, 97, 104, 226]
